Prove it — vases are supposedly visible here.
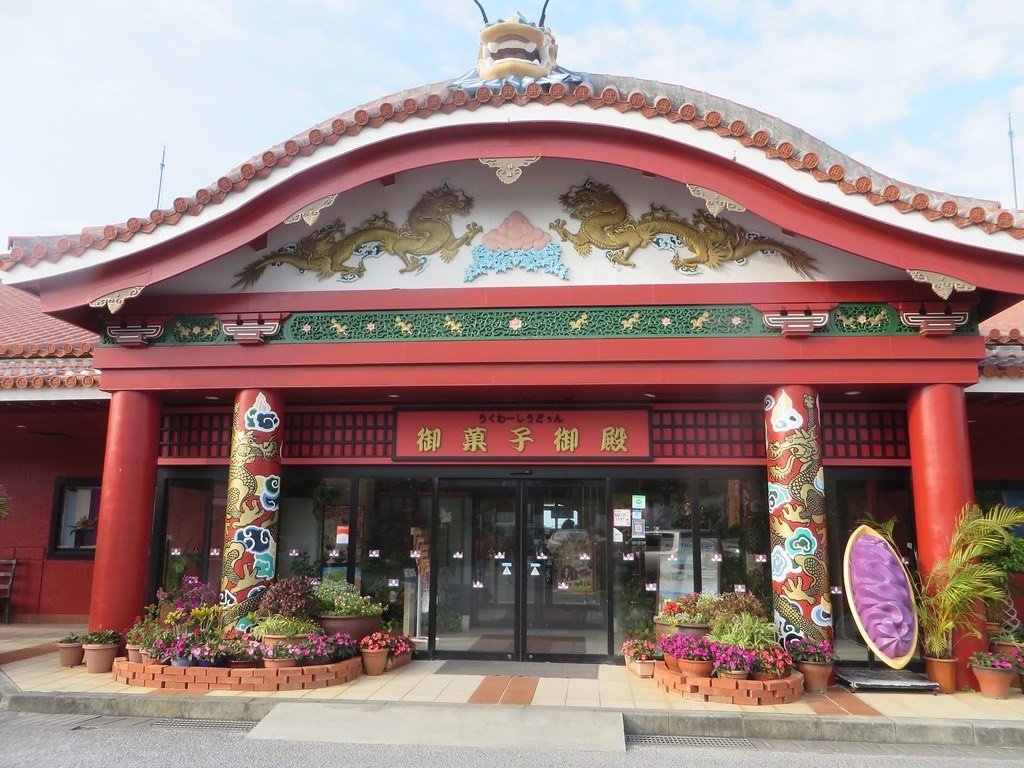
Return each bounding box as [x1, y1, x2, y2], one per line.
[263, 656, 296, 668]
[264, 634, 309, 645]
[663, 652, 681, 673]
[126, 643, 142, 662]
[362, 649, 389, 675]
[656, 620, 713, 645]
[717, 669, 749, 679]
[170, 650, 196, 667]
[74, 530, 87, 549]
[58, 630, 84, 667]
[677, 657, 712, 675]
[624, 654, 656, 678]
[200, 658, 222, 666]
[753, 667, 788, 680]
[796, 662, 834, 691]
[139, 649, 165, 664]
[228, 658, 255, 668]
[318, 616, 381, 644]
[384, 650, 412, 671]
[974, 664, 1018, 698]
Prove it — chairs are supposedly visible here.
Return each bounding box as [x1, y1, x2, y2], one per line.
[0, 559, 17, 624]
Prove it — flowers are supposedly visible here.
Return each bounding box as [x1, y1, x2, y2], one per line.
[124, 574, 420, 664]
[68, 516, 97, 535]
[615, 590, 840, 694]
[966, 650, 1024, 670]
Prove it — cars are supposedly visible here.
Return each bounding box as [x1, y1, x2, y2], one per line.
[542, 528, 593, 584]
[720, 536, 742, 560]
[626, 529, 724, 576]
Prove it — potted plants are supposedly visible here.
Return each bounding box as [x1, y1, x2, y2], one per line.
[846, 503, 1024, 695]
[82, 629, 123, 673]
[621, 574, 657, 621]
[988, 628, 1024, 654]
